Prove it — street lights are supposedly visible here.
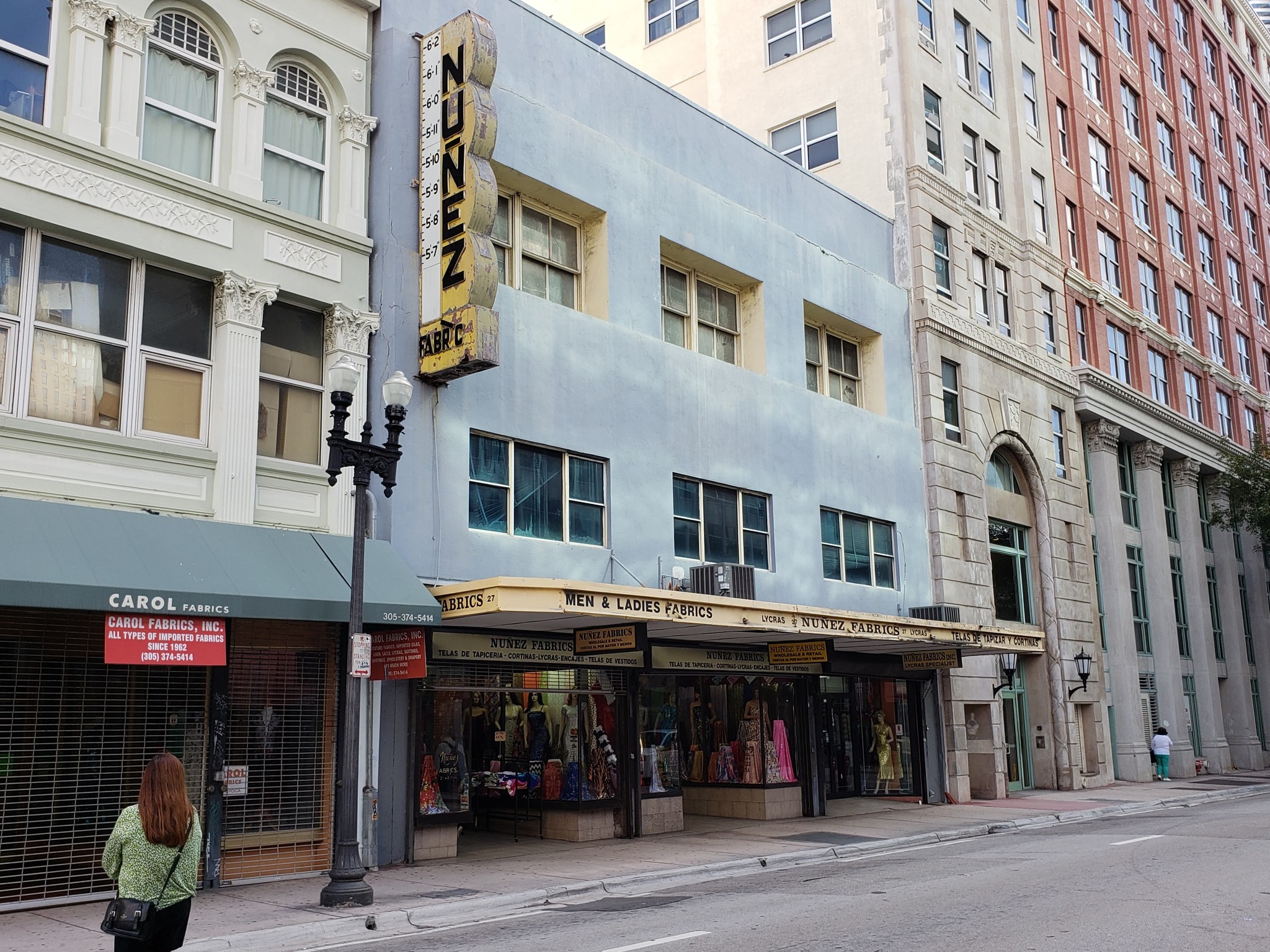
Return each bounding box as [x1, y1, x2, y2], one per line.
[318, 351, 414, 907]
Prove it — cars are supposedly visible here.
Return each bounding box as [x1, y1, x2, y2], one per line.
[612, 729, 677, 792]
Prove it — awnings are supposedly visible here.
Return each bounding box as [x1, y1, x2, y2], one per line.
[0, 496, 442, 625]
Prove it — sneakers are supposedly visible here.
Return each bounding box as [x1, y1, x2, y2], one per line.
[1163, 777, 1171, 781]
[1158, 775, 1162, 780]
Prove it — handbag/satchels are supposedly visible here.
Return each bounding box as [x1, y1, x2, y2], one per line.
[1150, 749, 1157, 763]
[100, 898, 155, 942]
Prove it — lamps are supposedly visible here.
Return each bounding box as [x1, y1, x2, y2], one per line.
[1068, 646, 1092, 700]
[992, 653, 1017, 699]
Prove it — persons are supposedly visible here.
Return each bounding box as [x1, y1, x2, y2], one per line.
[524, 692, 552, 761]
[1152, 727, 1173, 781]
[102, 754, 202, 952]
[638, 692, 648, 747]
[690, 689, 716, 747]
[432, 726, 468, 838]
[744, 687, 772, 742]
[653, 691, 681, 747]
[495, 692, 524, 763]
[869, 710, 894, 794]
[558, 686, 589, 767]
[462, 691, 490, 771]
